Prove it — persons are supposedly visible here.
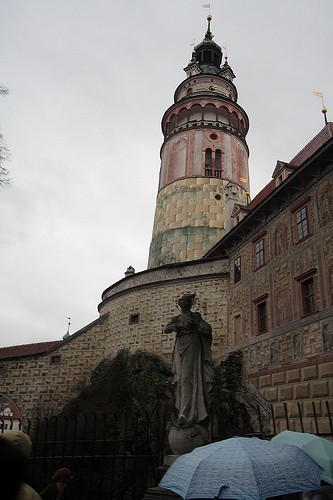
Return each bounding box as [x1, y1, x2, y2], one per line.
[0, 431, 76, 500]
[164, 293, 215, 426]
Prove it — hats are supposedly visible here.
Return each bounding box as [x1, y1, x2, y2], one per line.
[51, 467, 71, 482]
[0, 431, 32, 458]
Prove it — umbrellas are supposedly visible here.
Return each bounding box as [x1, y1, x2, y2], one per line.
[158, 430, 333, 500]
